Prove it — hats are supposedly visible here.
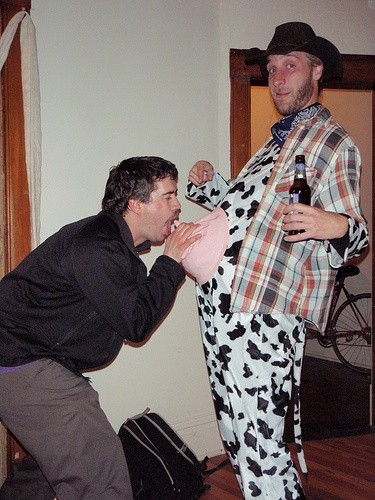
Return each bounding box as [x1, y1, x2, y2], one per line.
[172, 207, 229, 287]
[244, 21, 344, 84]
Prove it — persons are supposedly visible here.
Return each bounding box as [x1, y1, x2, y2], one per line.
[185, 22, 370, 500]
[0, 156, 202, 500]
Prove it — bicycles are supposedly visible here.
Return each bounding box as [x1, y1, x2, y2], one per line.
[296, 264, 372, 379]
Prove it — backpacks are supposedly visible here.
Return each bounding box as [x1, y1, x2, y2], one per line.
[117, 406, 211, 500]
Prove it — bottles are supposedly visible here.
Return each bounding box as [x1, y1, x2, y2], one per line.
[288, 155, 311, 235]
